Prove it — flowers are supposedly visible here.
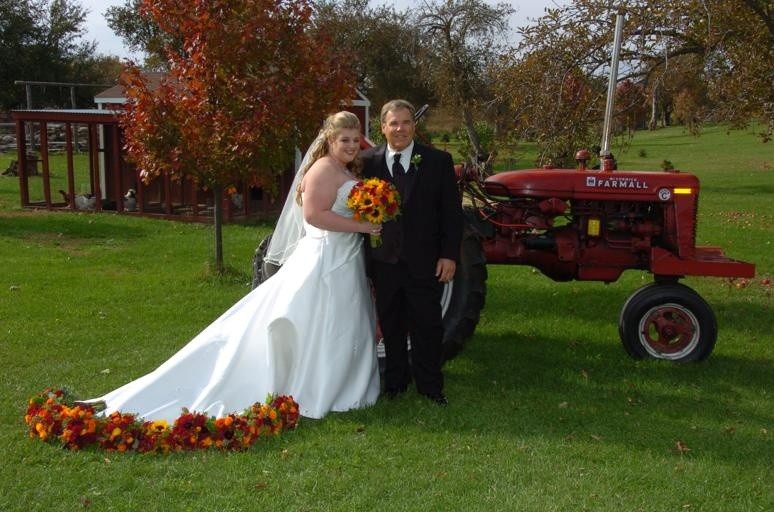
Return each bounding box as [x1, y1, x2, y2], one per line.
[411, 154, 421, 170]
[25, 387, 299, 453]
[346, 177, 403, 248]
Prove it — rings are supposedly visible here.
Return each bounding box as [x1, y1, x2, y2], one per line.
[373, 229, 376, 233]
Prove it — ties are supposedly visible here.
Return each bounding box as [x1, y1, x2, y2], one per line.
[392, 154, 405, 177]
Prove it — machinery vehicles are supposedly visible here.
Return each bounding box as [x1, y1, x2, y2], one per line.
[253, 105, 756, 380]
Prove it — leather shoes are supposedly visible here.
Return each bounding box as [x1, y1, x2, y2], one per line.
[427, 392, 448, 404]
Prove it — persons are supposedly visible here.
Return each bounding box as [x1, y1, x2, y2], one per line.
[352, 100, 464, 405]
[74, 111, 383, 427]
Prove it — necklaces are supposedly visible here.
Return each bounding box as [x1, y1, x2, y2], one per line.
[333, 157, 352, 177]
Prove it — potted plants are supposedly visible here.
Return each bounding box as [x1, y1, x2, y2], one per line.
[123, 189, 136, 211]
